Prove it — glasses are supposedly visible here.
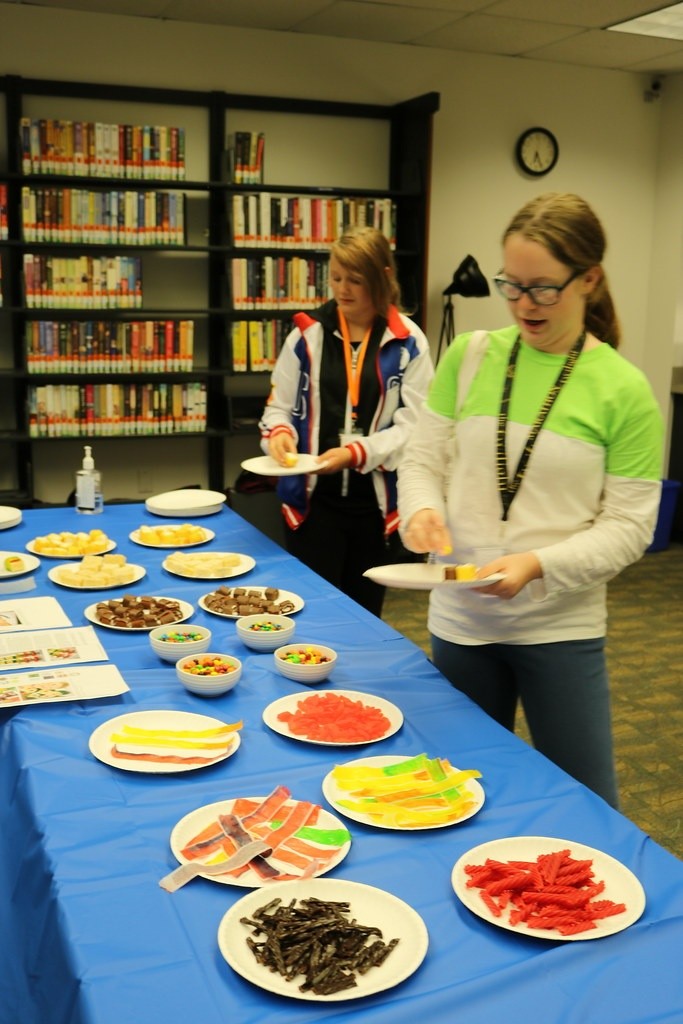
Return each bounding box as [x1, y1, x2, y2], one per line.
[494, 270, 579, 306]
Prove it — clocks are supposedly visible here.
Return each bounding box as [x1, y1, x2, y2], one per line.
[516, 127, 559, 177]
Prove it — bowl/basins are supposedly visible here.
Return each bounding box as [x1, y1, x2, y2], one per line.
[235, 614, 295, 652]
[175, 653, 242, 698]
[274, 644, 337, 684]
[149, 624, 211, 663]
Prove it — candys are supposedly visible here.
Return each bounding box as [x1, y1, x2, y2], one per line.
[183, 656, 237, 676]
[248, 622, 286, 631]
[280, 647, 332, 664]
[276, 693, 390, 743]
[158, 631, 204, 643]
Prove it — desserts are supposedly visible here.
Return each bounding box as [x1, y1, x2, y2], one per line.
[96, 594, 183, 628]
[204, 585, 294, 616]
[443, 563, 477, 581]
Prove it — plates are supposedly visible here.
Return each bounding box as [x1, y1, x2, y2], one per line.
[162, 552, 256, 579]
[0, 551, 40, 579]
[361, 563, 506, 591]
[145, 489, 227, 517]
[129, 525, 216, 548]
[451, 837, 646, 940]
[218, 878, 427, 1001]
[240, 454, 330, 477]
[83, 596, 194, 630]
[25, 538, 117, 558]
[322, 756, 485, 830]
[47, 562, 146, 589]
[170, 797, 351, 888]
[262, 690, 404, 746]
[0, 505, 21, 529]
[88, 710, 240, 773]
[197, 586, 304, 619]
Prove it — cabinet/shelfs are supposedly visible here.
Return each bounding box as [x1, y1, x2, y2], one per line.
[0, 74, 441, 508]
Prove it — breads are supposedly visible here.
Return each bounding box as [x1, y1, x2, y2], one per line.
[284, 452, 300, 468]
[167, 551, 242, 577]
[140, 523, 206, 546]
[58, 554, 135, 587]
[4, 556, 25, 571]
[34, 529, 111, 555]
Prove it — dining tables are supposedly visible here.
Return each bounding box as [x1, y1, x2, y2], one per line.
[0, 504, 683, 1024]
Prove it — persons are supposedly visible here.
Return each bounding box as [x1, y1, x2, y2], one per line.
[395, 194, 664, 813]
[258, 224, 436, 620]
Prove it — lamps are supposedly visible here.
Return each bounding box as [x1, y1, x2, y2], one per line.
[435, 255, 490, 367]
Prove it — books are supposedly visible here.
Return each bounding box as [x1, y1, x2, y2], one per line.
[1, 117, 397, 437]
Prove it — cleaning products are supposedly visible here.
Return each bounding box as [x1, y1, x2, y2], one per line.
[74, 445, 104, 516]
